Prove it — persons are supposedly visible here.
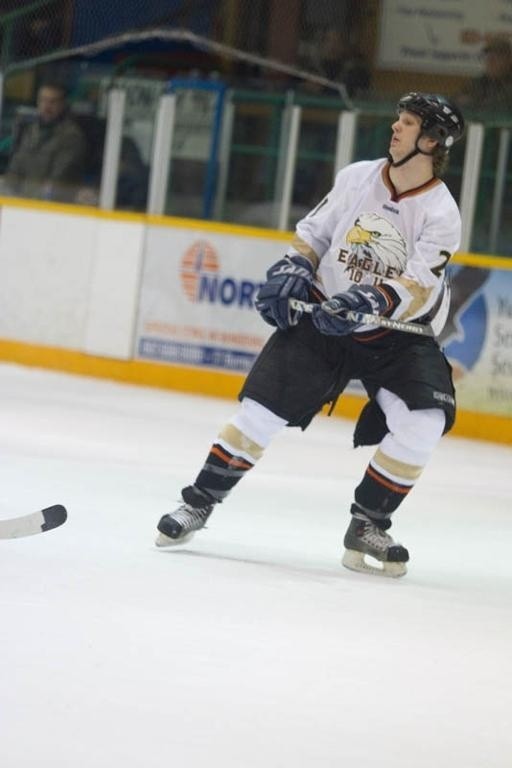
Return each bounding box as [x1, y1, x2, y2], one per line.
[0, 0, 512, 239]
[156, 86, 469, 562]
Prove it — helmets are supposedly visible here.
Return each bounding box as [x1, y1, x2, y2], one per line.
[397, 91, 464, 148]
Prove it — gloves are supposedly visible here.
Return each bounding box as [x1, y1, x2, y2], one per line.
[255, 253, 315, 331]
[311, 280, 395, 338]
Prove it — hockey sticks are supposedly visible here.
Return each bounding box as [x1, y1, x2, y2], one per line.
[291, 267, 451, 338]
[1, 503, 67, 541]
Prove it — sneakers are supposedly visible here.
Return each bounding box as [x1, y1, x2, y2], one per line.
[342, 500, 410, 564]
[157, 484, 215, 541]
